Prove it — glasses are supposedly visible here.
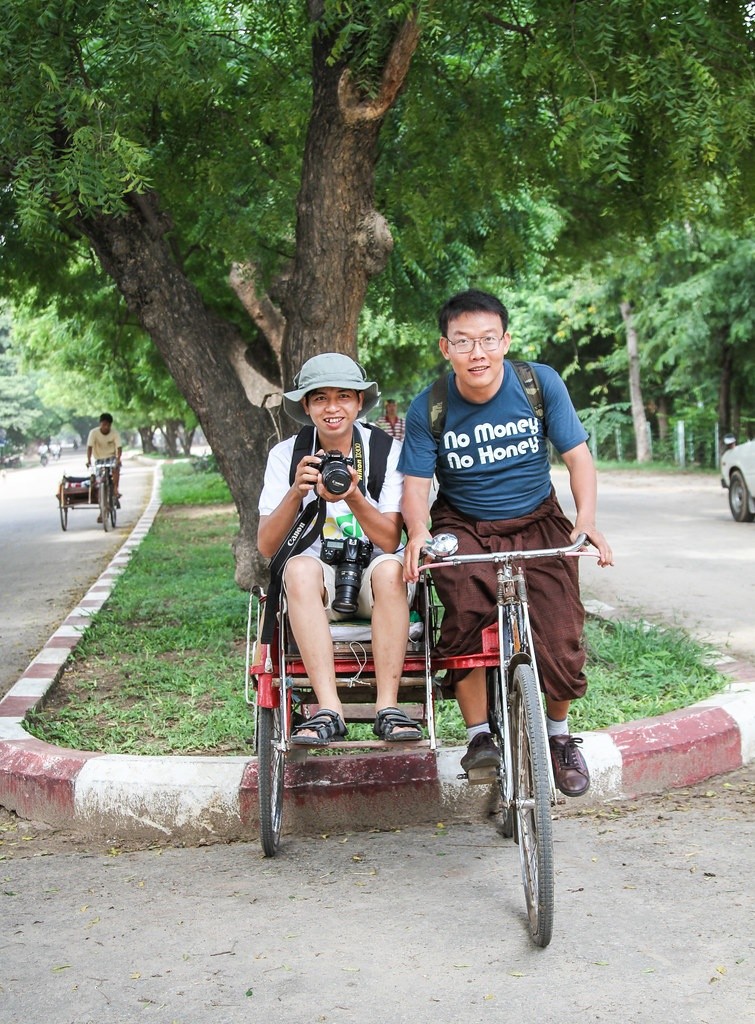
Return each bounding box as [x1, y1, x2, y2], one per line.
[448, 336, 504, 353]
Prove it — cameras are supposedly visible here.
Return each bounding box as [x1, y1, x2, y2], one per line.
[319, 536, 374, 614]
[306, 449, 356, 495]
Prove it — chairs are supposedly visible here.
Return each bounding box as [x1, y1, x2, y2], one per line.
[277, 581, 434, 664]
[62, 474, 94, 499]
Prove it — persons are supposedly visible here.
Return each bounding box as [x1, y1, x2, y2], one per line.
[396, 289, 616, 798]
[86, 412, 123, 524]
[375, 399, 406, 442]
[256, 352, 425, 747]
[36, 442, 49, 455]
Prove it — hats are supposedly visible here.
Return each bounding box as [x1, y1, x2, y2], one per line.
[283, 353, 381, 426]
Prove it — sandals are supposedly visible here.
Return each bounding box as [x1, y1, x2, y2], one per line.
[291, 709, 349, 744]
[373, 707, 421, 741]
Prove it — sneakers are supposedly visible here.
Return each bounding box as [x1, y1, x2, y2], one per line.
[461, 732, 501, 771]
[549, 735, 590, 797]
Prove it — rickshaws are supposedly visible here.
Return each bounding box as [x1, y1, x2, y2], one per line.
[239, 530, 615, 949]
[55, 456, 123, 532]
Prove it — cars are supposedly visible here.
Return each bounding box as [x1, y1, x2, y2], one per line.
[719, 430, 755, 523]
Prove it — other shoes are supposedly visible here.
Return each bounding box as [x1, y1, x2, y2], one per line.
[97, 516, 102, 523]
